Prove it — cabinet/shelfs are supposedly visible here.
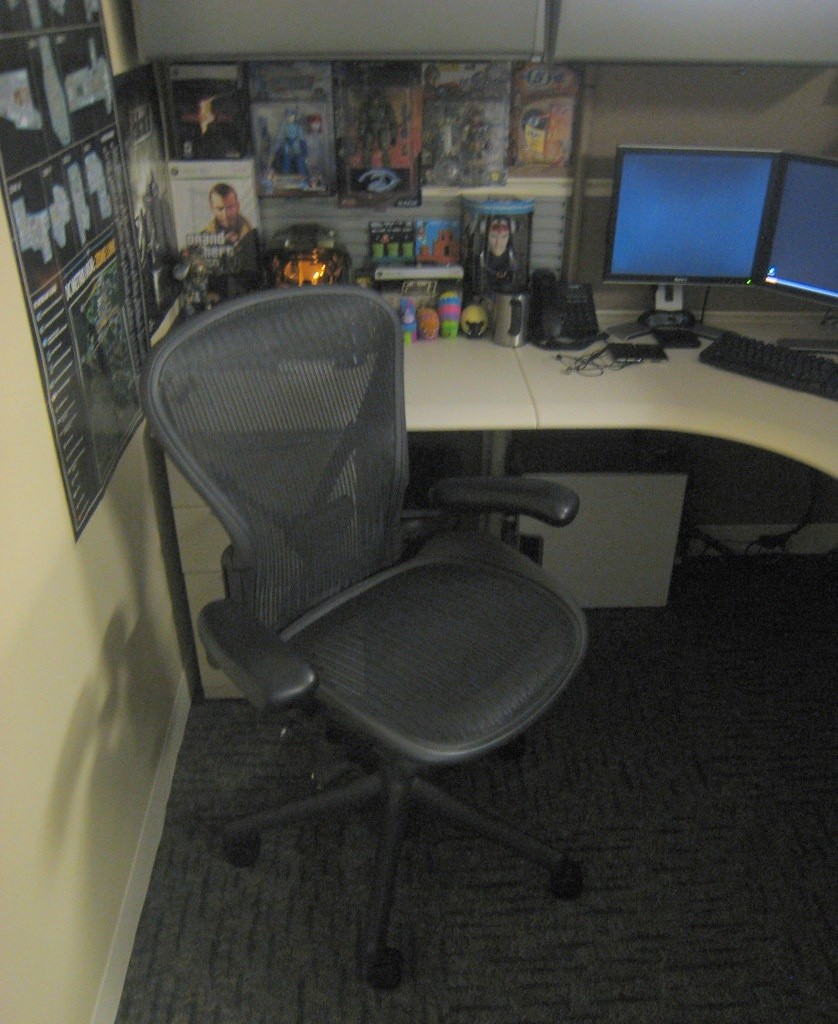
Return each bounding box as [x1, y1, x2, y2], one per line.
[129, 0, 838, 67]
[148, 337, 539, 702]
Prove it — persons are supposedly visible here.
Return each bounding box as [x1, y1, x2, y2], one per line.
[272, 108, 310, 176]
[476, 216, 519, 288]
[359, 89, 398, 173]
[192, 181, 252, 273]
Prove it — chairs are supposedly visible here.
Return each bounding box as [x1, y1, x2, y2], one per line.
[139, 283, 588, 990]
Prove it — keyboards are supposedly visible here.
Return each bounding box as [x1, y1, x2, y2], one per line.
[699, 330, 838, 403]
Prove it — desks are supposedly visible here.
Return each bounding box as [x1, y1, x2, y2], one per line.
[516, 313, 838, 483]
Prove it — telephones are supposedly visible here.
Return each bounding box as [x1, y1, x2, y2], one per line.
[529, 268, 600, 343]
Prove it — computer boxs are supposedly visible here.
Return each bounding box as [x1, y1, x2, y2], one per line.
[512, 444, 690, 609]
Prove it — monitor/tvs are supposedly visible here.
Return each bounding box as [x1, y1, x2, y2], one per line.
[601, 144, 783, 343]
[756, 150, 838, 356]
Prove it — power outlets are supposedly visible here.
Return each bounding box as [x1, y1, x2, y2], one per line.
[740, 524, 789, 555]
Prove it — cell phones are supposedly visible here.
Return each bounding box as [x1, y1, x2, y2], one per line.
[611, 343, 668, 362]
